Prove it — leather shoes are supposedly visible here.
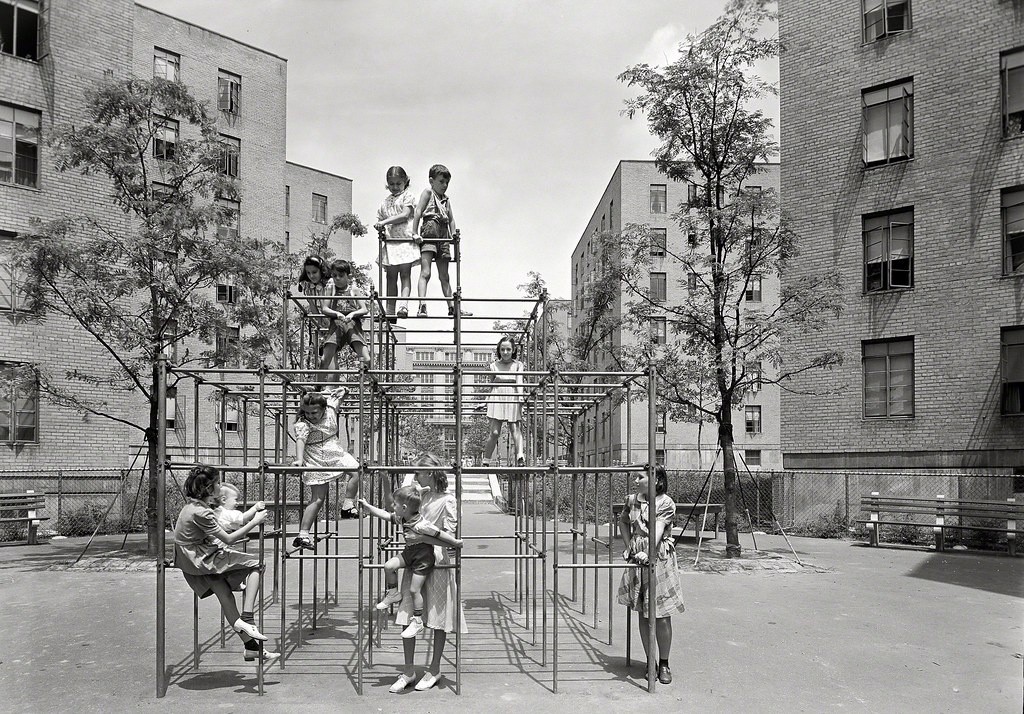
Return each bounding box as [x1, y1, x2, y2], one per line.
[243, 648, 281, 661]
[233, 618, 268, 641]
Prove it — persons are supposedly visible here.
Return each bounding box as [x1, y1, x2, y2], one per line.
[388, 453, 468, 693]
[357, 486, 463, 639]
[481, 337, 526, 467]
[374, 165, 422, 324]
[173, 465, 281, 661]
[292, 386, 367, 550]
[616, 460, 686, 684]
[299, 254, 340, 395]
[210, 483, 264, 591]
[305, 260, 379, 393]
[412, 164, 473, 318]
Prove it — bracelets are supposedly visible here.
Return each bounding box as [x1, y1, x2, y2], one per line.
[342, 386, 347, 393]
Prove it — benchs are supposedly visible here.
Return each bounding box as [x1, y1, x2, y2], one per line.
[855, 491, 1024, 557]
[0, 489, 49, 545]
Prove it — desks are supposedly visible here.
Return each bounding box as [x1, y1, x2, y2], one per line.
[608, 503, 724, 544]
[238, 501, 309, 530]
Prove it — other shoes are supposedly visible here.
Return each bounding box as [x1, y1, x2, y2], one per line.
[645, 668, 658, 681]
[400, 621, 425, 639]
[517, 457, 525, 467]
[659, 667, 672, 683]
[376, 591, 403, 609]
[483, 458, 490, 465]
[417, 304, 427, 315]
[292, 537, 314, 550]
[448, 306, 473, 316]
[341, 507, 366, 518]
[374, 317, 397, 324]
[397, 306, 408, 319]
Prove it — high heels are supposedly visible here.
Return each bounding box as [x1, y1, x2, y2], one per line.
[415, 671, 441, 691]
[389, 671, 416, 693]
